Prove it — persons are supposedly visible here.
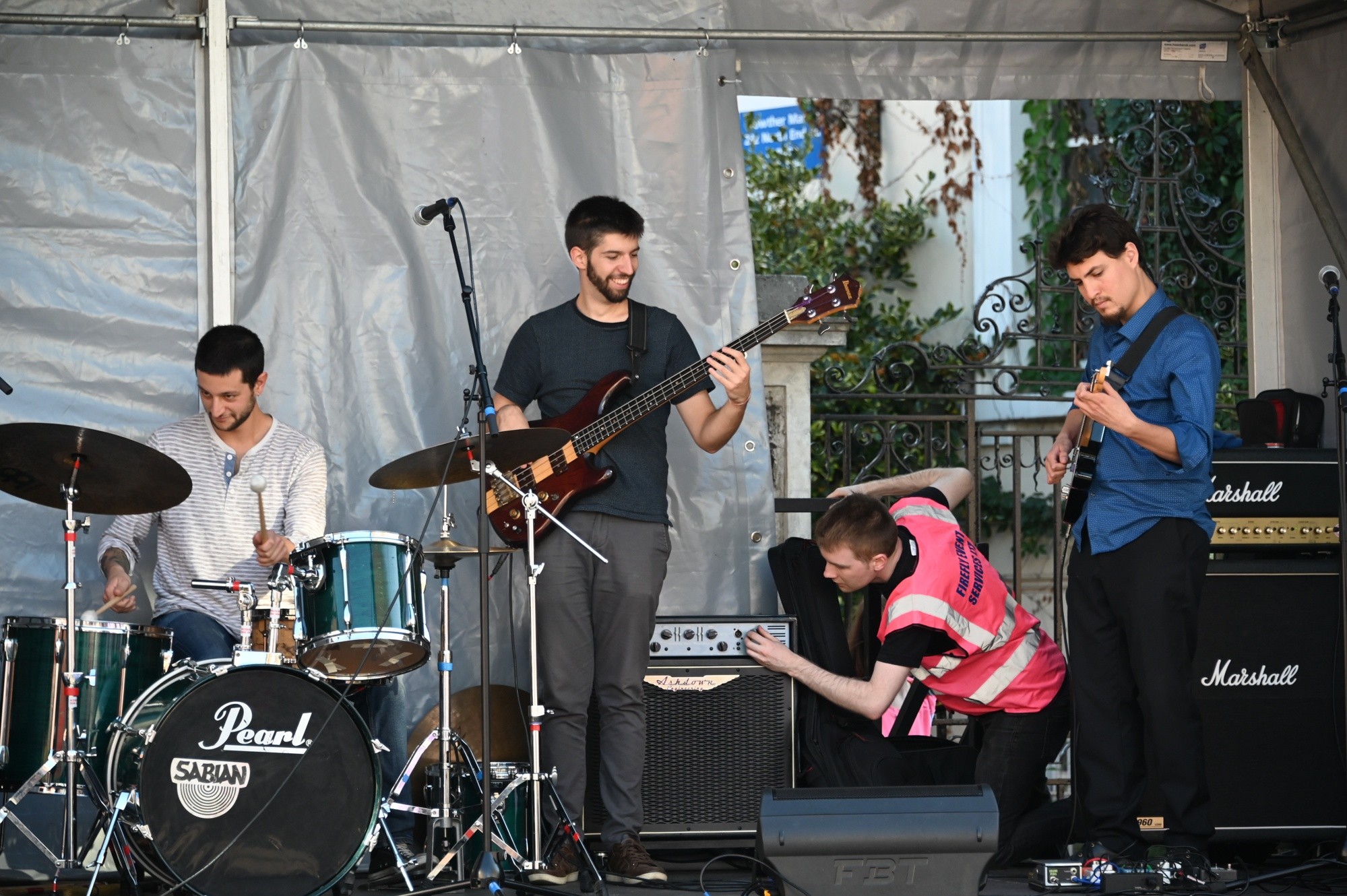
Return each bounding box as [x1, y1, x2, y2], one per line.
[95, 324, 417, 874]
[1044, 204, 1222, 864]
[485, 194, 752, 885]
[744, 468, 1070, 879]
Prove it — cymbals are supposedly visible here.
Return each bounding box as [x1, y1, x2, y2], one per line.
[424, 540, 520, 565]
[0, 422, 193, 516]
[368, 427, 571, 490]
[407, 684, 530, 792]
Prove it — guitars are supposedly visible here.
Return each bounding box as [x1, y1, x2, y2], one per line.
[1063, 360, 1112, 526]
[486, 271, 862, 546]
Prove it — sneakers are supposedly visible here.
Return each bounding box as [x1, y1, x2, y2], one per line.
[368, 842, 438, 886]
[606, 838, 669, 884]
[528, 841, 579, 884]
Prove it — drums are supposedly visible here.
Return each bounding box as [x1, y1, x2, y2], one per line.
[289, 529, 431, 680]
[241, 608, 297, 668]
[0, 614, 175, 797]
[106, 661, 383, 896]
[424, 761, 531, 872]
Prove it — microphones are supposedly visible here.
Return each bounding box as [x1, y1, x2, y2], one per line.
[413, 197, 458, 226]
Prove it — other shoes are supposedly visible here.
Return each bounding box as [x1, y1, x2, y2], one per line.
[1159, 846, 1199, 890]
[1068, 842, 1123, 869]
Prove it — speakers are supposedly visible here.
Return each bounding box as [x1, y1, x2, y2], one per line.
[581, 657, 797, 847]
[753, 783, 999, 896]
[1185, 554, 1347, 841]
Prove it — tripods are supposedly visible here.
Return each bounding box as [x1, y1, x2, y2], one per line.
[354, 211, 609, 896]
[0, 453, 146, 896]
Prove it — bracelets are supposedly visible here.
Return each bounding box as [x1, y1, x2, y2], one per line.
[729, 388, 751, 405]
